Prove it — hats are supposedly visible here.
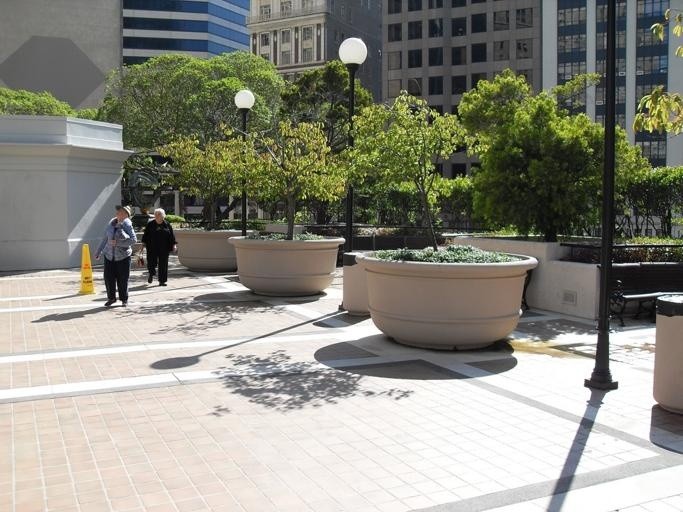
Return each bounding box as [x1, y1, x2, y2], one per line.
[115, 204, 136, 220]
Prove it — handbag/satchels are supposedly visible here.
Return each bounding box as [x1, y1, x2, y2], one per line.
[137, 257, 145, 268]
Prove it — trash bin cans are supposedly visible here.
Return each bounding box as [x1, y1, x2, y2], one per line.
[652, 294, 683, 414]
[342, 252, 371, 316]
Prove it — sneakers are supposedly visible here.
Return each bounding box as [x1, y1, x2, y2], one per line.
[122, 299, 127, 306]
[105, 298, 116, 306]
[159, 281, 167, 286]
[148, 275, 153, 283]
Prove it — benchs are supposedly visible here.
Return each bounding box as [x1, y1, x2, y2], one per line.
[595, 261, 682, 328]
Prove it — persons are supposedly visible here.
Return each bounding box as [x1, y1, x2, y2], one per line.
[94, 205, 137, 307]
[138, 208, 177, 286]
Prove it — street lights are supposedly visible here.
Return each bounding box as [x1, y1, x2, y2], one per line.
[334, 33, 375, 252]
[230, 89, 258, 239]
